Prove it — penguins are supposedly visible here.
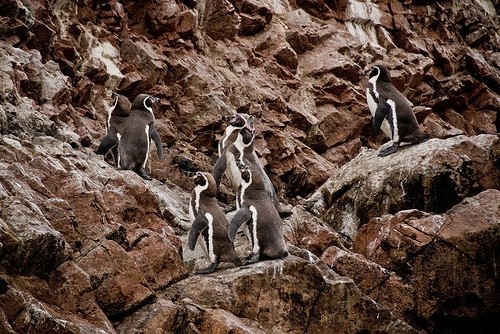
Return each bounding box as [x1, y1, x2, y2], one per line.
[212, 126, 293, 217]
[93, 93, 131, 167]
[226, 163, 289, 266]
[115, 93, 163, 181]
[366, 63, 431, 158]
[217, 114, 248, 160]
[186, 171, 242, 274]
